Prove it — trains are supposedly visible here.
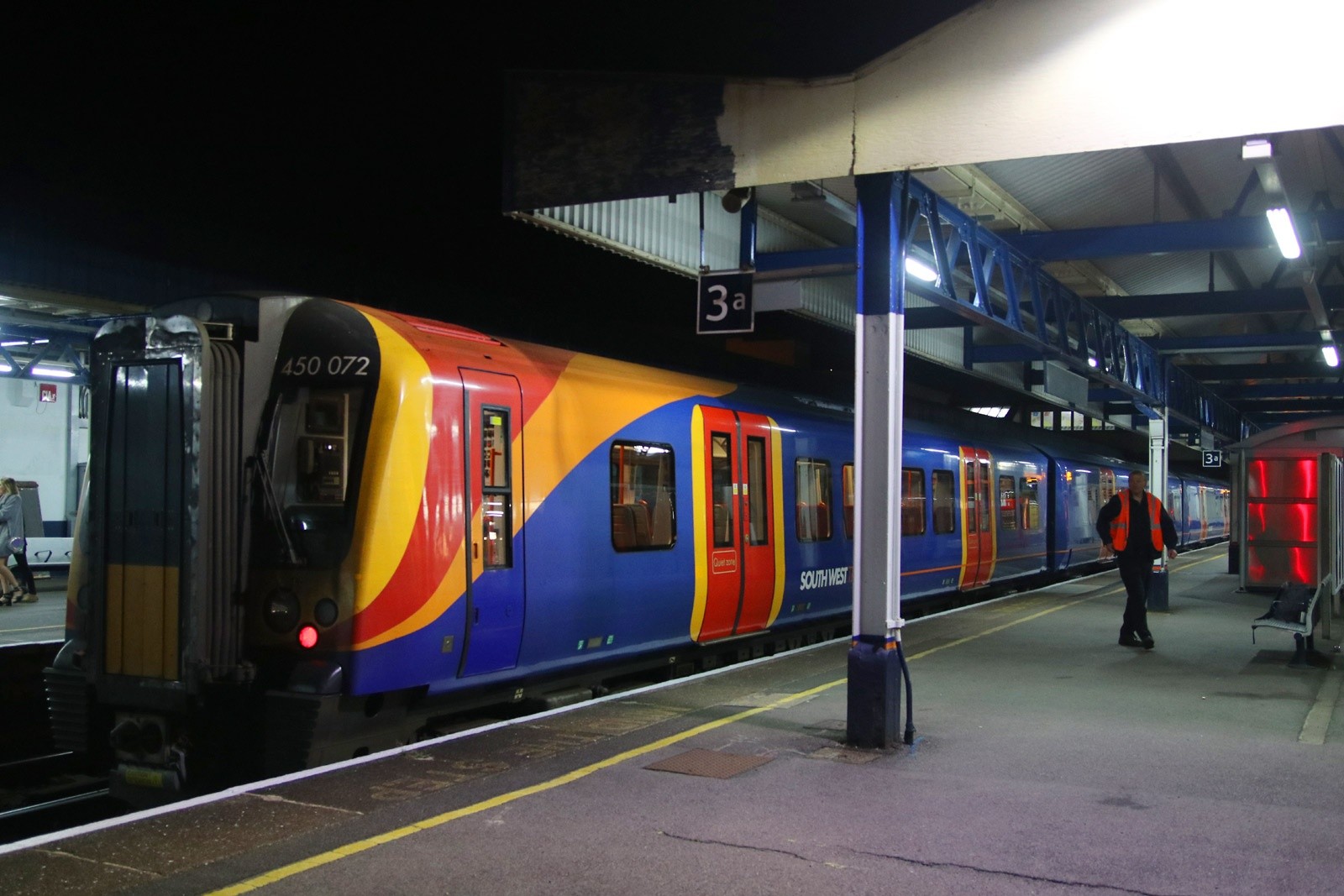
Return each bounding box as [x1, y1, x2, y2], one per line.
[0, 348, 91, 576]
[41, 292, 1231, 793]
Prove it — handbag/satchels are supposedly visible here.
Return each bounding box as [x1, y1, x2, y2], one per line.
[6, 537, 24, 555]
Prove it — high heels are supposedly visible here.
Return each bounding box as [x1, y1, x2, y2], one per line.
[0, 593, 12, 606]
[12, 587, 25, 603]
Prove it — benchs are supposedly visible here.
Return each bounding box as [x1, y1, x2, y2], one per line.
[1251, 584, 1323, 667]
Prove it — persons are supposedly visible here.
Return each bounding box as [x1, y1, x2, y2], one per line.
[1096, 472, 1180, 650]
[0, 476, 39, 607]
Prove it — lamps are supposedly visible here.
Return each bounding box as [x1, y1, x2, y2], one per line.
[720, 187, 752, 214]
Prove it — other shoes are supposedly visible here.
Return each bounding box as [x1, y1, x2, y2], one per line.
[21, 594, 39, 602]
[1141, 635, 1155, 650]
[1118, 636, 1142, 647]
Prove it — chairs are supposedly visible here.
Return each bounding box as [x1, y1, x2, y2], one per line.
[611, 502, 853, 548]
[901, 506, 956, 533]
